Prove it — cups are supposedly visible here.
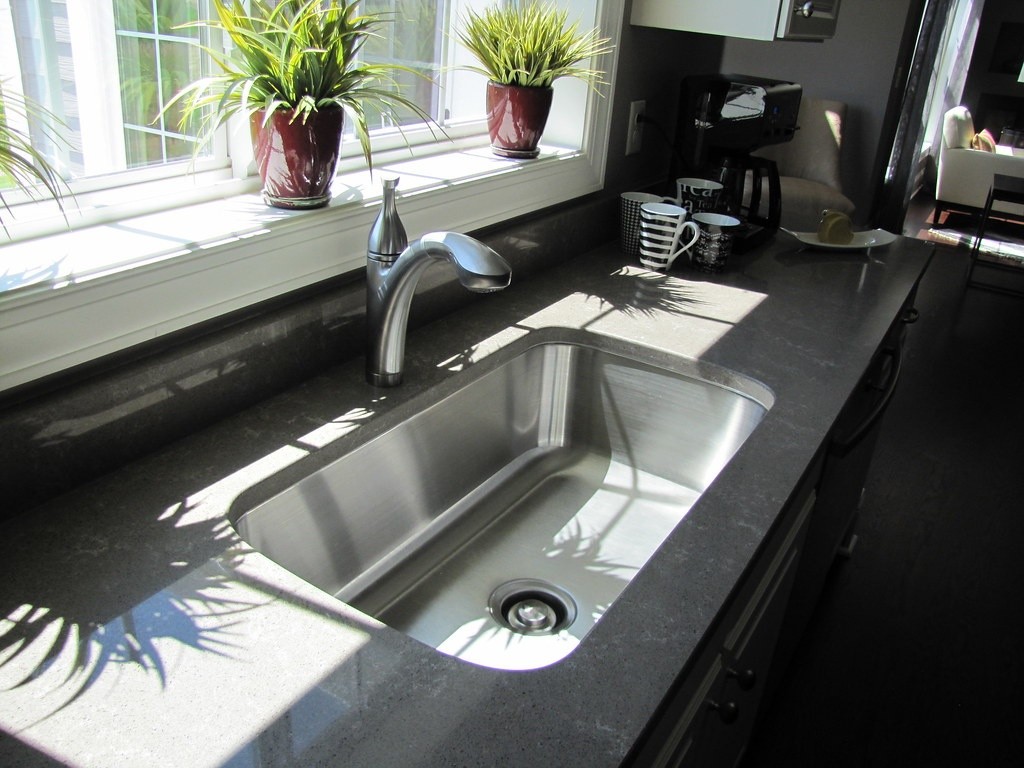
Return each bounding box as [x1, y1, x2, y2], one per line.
[639, 203, 700, 272]
[621, 191, 676, 254]
[676, 178, 723, 238]
[692, 212, 740, 272]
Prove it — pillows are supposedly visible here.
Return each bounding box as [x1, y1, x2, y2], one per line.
[972, 133, 980, 150]
[978, 128, 995, 153]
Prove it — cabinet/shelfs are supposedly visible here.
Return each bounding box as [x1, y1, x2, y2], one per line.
[630, 0, 841, 43]
[637, 287, 922, 768]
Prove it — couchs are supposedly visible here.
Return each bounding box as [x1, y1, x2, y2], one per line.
[741, 99, 856, 230]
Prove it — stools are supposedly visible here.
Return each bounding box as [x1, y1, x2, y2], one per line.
[961, 174, 1024, 305]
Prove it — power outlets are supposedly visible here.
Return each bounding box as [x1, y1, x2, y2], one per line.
[625, 100, 645, 156]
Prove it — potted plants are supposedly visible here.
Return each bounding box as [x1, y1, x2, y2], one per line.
[433, 0, 617, 159]
[148, 0, 452, 209]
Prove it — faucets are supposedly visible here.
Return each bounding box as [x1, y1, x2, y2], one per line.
[358, 172, 515, 391]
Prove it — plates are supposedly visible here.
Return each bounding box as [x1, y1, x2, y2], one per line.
[779, 227, 898, 248]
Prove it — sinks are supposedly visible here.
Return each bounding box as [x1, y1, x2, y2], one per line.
[220, 321, 778, 675]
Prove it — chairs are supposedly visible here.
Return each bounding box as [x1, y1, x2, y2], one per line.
[932, 106, 1023, 228]
